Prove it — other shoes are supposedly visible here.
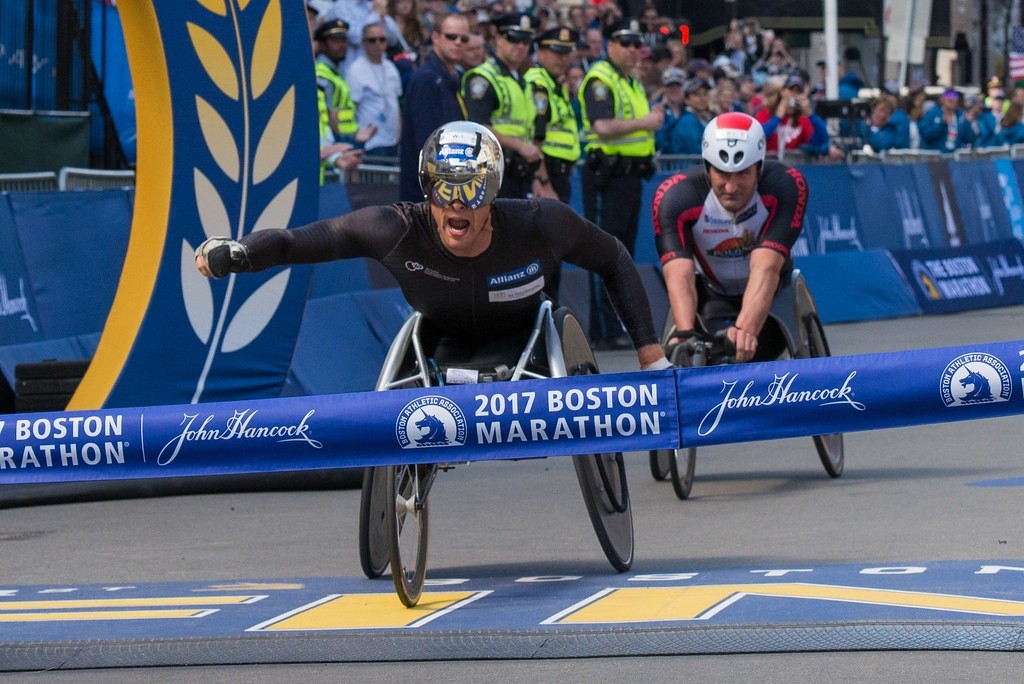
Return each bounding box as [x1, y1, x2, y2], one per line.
[590, 334, 633, 352]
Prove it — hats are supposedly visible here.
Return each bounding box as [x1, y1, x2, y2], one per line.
[780, 74, 807, 91]
[489, 11, 542, 40]
[659, 65, 686, 85]
[681, 75, 715, 95]
[602, 19, 648, 43]
[313, 18, 349, 40]
[540, 27, 578, 52]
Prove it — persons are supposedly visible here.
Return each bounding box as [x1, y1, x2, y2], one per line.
[654, 112, 810, 362]
[838, 61, 1024, 155]
[305, 0, 846, 172]
[529, 26, 583, 301]
[195, 122, 679, 370]
[461, 14, 543, 200]
[579, 19, 665, 349]
[399, 12, 470, 200]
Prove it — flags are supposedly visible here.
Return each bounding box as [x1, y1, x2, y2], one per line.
[1008, 25, 1024, 81]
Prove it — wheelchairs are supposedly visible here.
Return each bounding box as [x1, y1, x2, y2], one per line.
[646, 267, 846, 500]
[358, 297, 635, 611]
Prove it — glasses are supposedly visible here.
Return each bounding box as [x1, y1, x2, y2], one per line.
[445, 33, 469, 43]
[504, 33, 530, 45]
[369, 37, 387, 43]
[619, 39, 642, 49]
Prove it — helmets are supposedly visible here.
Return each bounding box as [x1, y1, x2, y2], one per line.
[417, 122, 506, 210]
[699, 112, 767, 171]
